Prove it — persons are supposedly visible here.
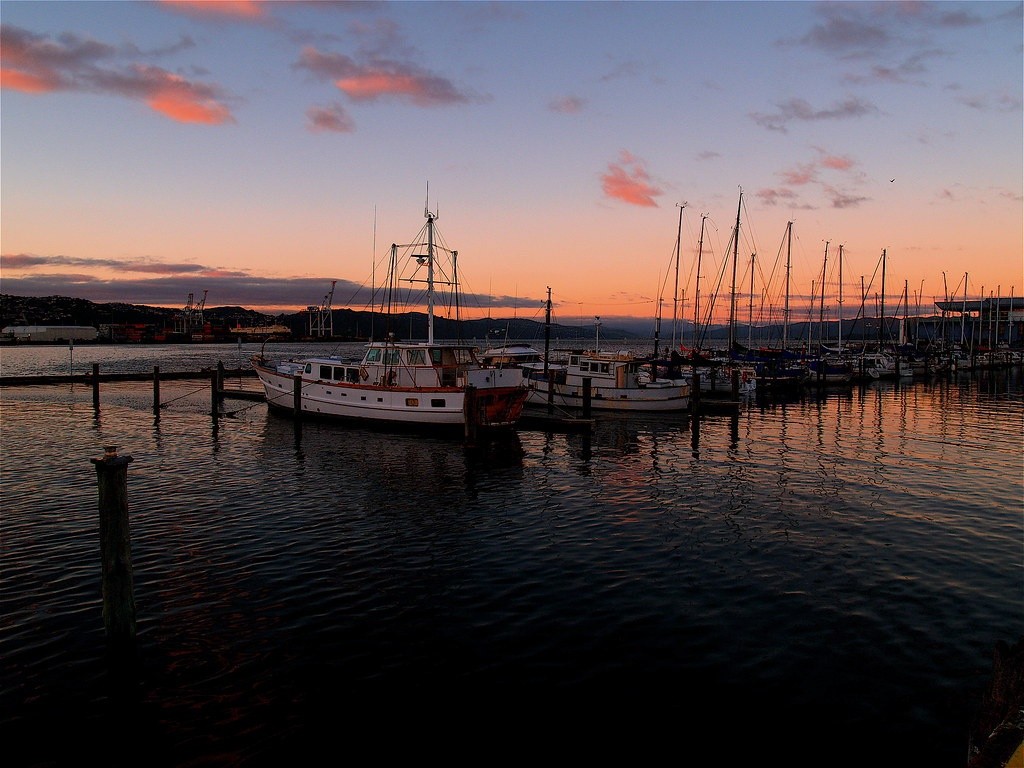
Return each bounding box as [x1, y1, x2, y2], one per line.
[665, 346, 669, 353]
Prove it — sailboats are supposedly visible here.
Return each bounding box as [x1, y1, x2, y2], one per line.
[248, 181, 1024, 436]
